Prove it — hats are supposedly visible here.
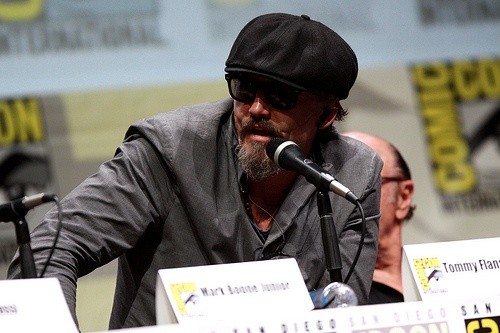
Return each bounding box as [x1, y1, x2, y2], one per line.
[224, 12, 359, 100]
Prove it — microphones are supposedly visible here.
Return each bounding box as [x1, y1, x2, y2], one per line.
[0, 191, 58, 225]
[266, 138, 359, 207]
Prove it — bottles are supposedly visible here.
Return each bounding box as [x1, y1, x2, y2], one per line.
[307, 282, 356, 310]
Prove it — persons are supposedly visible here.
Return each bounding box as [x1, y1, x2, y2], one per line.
[339, 132, 417, 305]
[7, 13, 383, 332]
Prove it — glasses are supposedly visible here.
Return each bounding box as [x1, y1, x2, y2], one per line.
[225, 74, 317, 110]
